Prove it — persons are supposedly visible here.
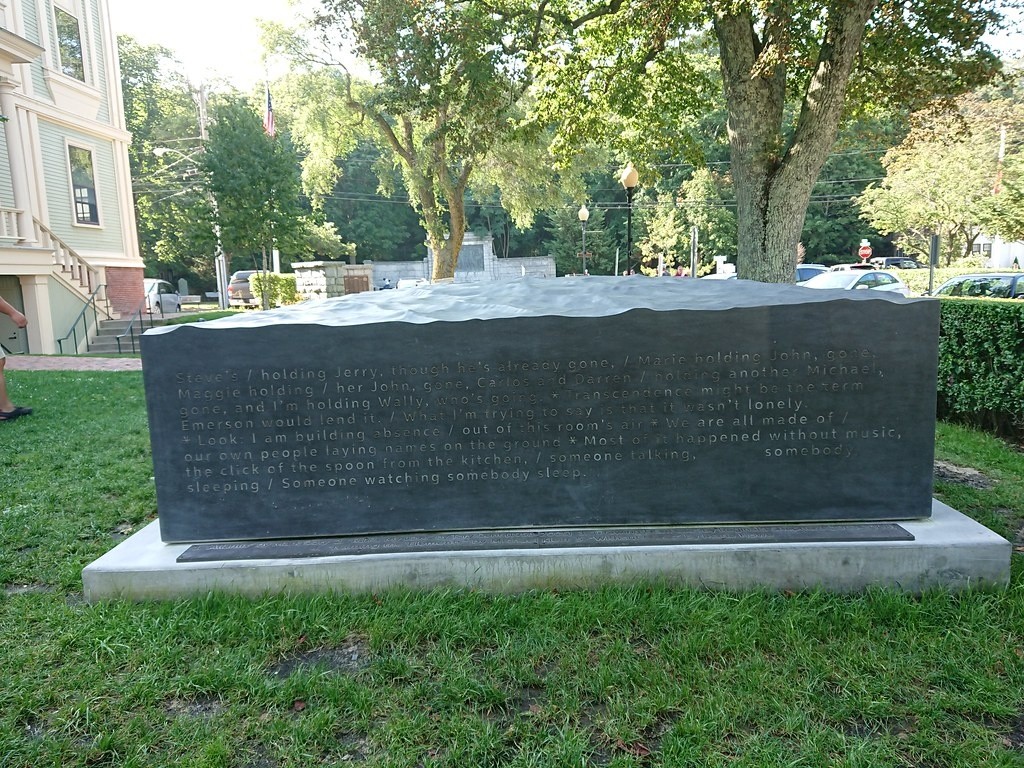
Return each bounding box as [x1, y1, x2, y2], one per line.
[623, 266, 684, 276]
[175, 290, 182, 312]
[0, 296, 34, 420]
[383, 278, 391, 289]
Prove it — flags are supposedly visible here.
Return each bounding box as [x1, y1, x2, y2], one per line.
[265, 82, 274, 135]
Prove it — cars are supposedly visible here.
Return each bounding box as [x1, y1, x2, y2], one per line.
[143, 278, 183, 315]
[804, 270, 911, 299]
[831, 263, 877, 273]
[395, 277, 429, 288]
[921, 272, 1024, 300]
[700, 273, 739, 280]
[793, 264, 833, 287]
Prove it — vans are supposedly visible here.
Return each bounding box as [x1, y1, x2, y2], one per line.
[869, 256, 927, 270]
[227, 270, 270, 307]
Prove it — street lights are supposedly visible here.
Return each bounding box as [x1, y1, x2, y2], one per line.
[577, 202, 590, 277]
[620, 162, 640, 276]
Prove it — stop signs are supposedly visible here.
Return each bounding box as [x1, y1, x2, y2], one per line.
[859, 246, 872, 259]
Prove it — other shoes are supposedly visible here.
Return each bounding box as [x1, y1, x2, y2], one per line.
[0, 407, 33, 421]
